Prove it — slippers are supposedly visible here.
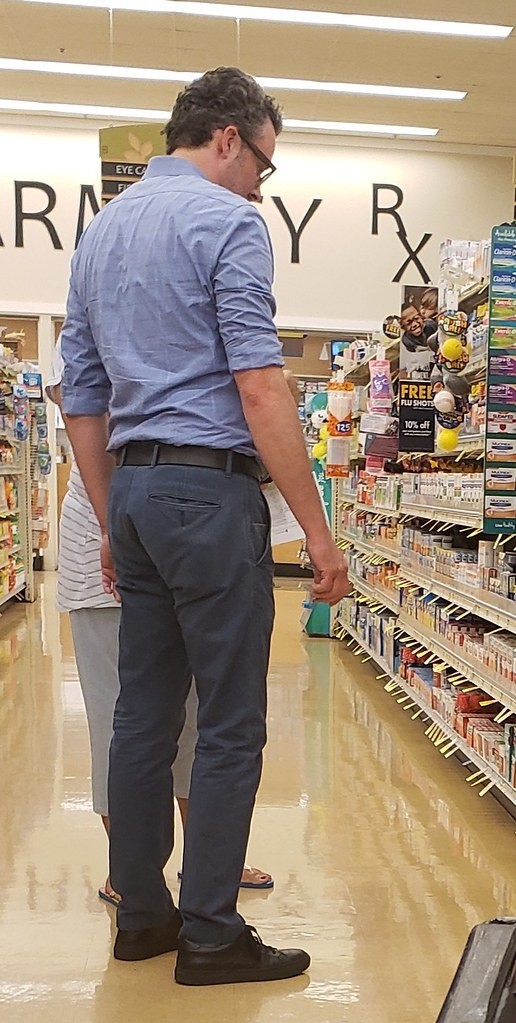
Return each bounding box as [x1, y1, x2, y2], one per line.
[98, 886, 121, 908]
[178, 862, 274, 888]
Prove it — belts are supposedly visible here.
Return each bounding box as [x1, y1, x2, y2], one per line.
[114, 442, 262, 481]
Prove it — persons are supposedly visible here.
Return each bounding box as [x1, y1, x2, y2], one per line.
[56, 67, 353, 985]
[401, 288, 437, 350]
[45, 331, 279, 907]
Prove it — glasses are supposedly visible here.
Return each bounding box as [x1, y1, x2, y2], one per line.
[218, 124, 277, 186]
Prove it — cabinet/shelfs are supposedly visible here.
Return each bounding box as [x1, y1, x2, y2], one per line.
[0, 337, 58, 617]
[326, 277, 516, 821]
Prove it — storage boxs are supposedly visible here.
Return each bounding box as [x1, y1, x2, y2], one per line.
[339, 472, 516, 789]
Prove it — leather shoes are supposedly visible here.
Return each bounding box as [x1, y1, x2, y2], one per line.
[115, 909, 182, 961]
[175, 924, 309, 985]
